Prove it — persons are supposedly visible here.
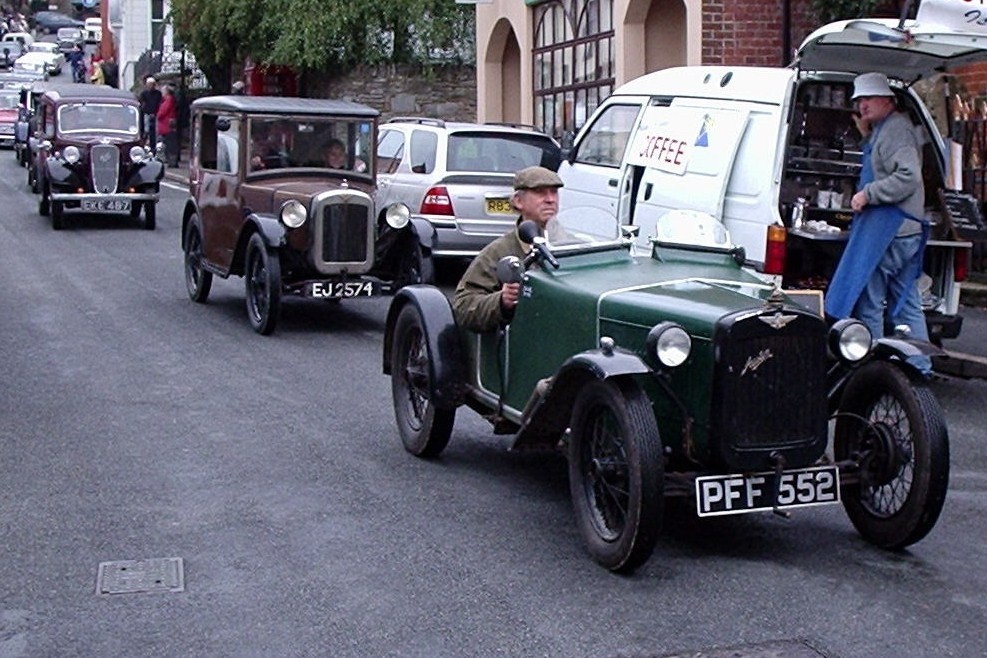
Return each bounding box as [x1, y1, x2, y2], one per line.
[826, 72, 936, 383]
[137, 77, 348, 170]
[453, 167, 593, 334]
[68, 45, 120, 89]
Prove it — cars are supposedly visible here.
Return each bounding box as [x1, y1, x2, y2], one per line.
[0, 10, 103, 192]
[379, 206, 949, 576]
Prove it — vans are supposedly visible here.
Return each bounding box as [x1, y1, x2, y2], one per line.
[558, 14, 986, 358]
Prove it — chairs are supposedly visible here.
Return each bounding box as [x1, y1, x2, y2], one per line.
[497, 156, 529, 173]
[423, 148, 458, 174]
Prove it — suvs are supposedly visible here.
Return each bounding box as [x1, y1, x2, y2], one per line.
[373, 115, 564, 288]
[179, 95, 437, 337]
[29, 85, 168, 230]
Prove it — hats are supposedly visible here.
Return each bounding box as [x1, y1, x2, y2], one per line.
[851, 72, 895, 99]
[514, 165, 564, 190]
